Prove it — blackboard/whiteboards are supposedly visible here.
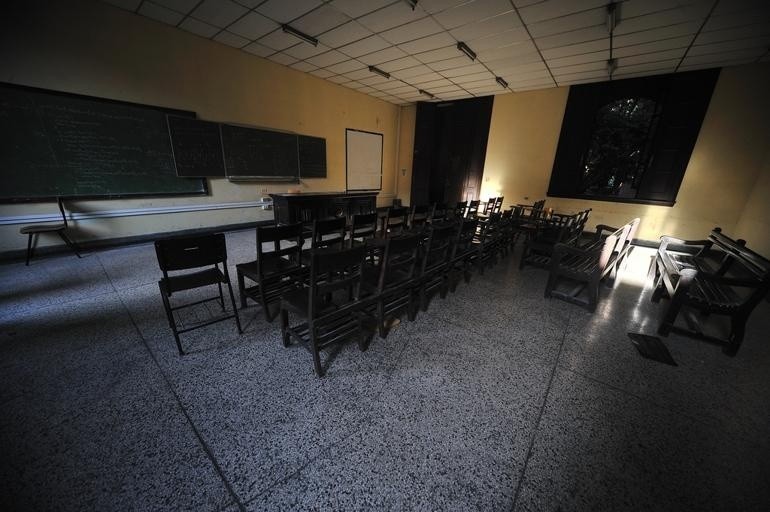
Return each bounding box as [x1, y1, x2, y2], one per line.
[0, 81, 209, 203]
[166, 115, 327, 179]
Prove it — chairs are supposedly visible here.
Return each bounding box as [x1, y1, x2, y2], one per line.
[513, 206, 525, 243]
[466, 200, 480, 216]
[347, 210, 376, 243]
[486, 213, 509, 268]
[410, 202, 429, 232]
[431, 200, 448, 222]
[481, 196, 495, 214]
[410, 223, 451, 309]
[515, 201, 542, 246]
[282, 243, 367, 378]
[154, 231, 243, 357]
[304, 214, 346, 301]
[494, 197, 504, 213]
[237, 222, 305, 320]
[505, 209, 514, 254]
[447, 219, 481, 294]
[524, 199, 547, 241]
[454, 201, 467, 217]
[376, 205, 408, 240]
[466, 218, 503, 276]
[357, 233, 419, 338]
[20, 195, 81, 266]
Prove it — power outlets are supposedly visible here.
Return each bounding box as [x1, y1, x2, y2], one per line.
[262, 197, 271, 203]
[262, 205, 269, 209]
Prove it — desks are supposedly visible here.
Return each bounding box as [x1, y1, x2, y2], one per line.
[268, 192, 379, 241]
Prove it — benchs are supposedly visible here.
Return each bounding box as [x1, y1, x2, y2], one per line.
[544, 218, 640, 312]
[518, 207, 592, 269]
[647, 228, 770, 357]
